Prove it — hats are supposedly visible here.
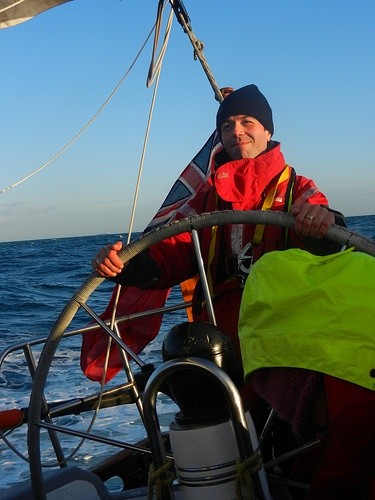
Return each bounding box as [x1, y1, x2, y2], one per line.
[216, 84, 274, 145]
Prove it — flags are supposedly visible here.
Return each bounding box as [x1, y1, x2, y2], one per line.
[80, 129, 225, 384]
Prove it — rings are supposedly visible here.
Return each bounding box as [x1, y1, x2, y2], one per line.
[306, 215, 314, 219]
[96, 259, 101, 264]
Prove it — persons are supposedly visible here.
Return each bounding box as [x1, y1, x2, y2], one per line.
[90, 84, 348, 472]
[237, 247, 375, 499]
